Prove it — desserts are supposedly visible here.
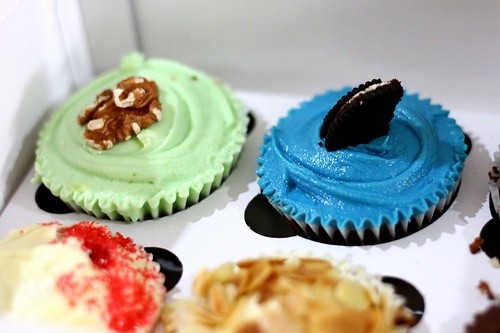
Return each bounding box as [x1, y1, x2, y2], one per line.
[160, 254, 405, 333]
[0, 220, 167, 333]
[35, 50, 249, 223]
[254, 78, 467, 246]
